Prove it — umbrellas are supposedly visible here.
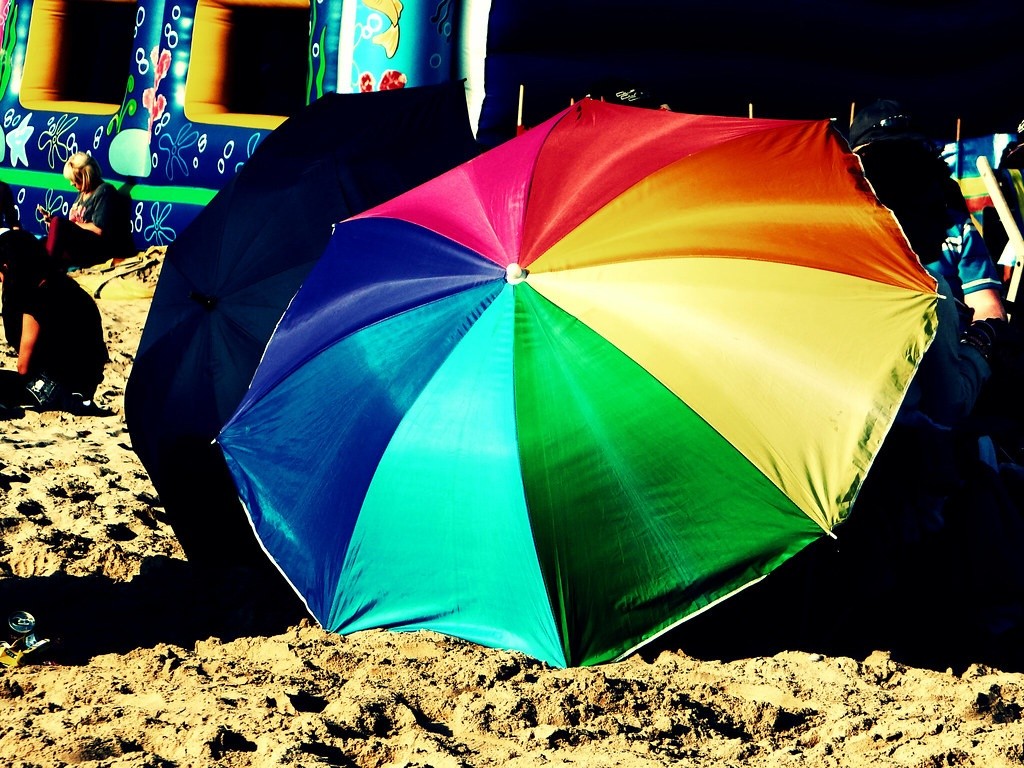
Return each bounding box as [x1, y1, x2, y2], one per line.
[116, 78, 944, 675]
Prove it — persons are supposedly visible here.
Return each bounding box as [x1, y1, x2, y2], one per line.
[842, 97, 1012, 431]
[0, 151, 121, 409]
